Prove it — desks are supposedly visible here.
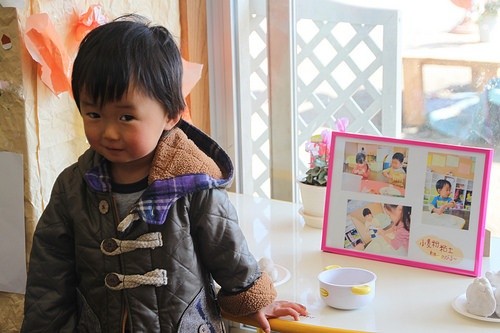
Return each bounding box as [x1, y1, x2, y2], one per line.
[402, 42, 500, 127]
[227, 192, 500, 333]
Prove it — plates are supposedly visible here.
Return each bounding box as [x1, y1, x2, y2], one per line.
[271, 264, 291, 287]
[450, 293, 500, 323]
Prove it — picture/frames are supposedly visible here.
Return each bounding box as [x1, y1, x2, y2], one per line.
[321, 130, 495, 277]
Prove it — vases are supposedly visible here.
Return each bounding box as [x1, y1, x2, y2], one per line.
[298, 181, 327, 230]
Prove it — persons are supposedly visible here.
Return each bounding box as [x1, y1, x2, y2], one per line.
[20, 21, 308, 333]
[362, 208, 380, 238]
[382, 152, 406, 188]
[382, 203, 412, 255]
[352, 153, 371, 180]
[429, 180, 456, 215]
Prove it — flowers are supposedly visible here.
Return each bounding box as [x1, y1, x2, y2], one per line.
[305, 131, 332, 186]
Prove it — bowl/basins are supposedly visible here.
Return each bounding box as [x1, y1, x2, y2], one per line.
[317, 264, 378, 311]
